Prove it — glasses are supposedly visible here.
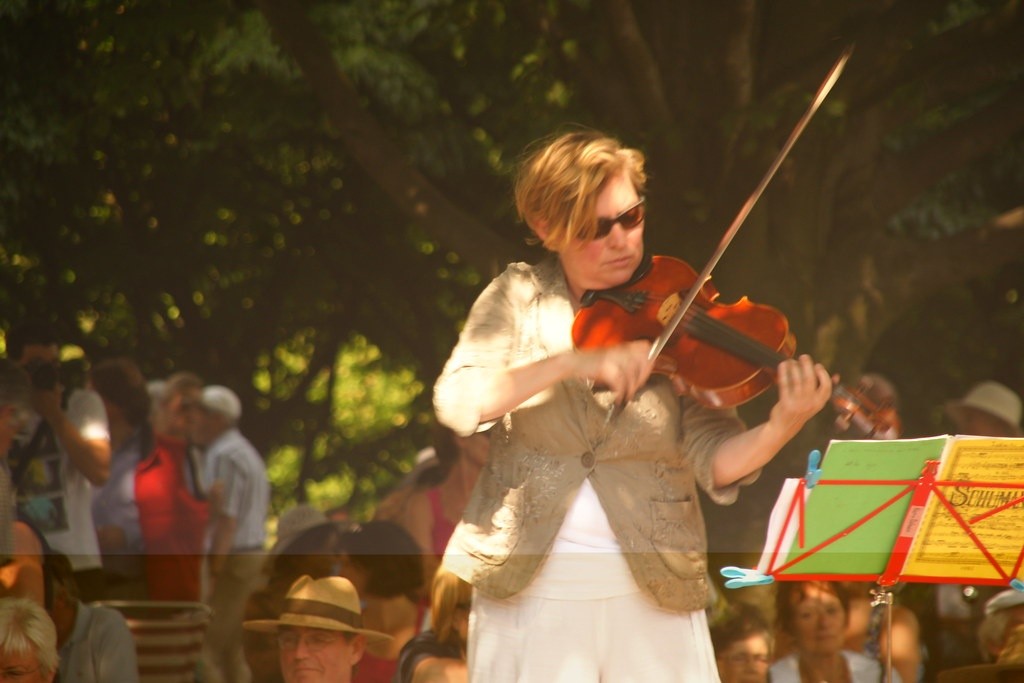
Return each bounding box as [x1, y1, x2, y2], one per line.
[567, 196, 648, 240]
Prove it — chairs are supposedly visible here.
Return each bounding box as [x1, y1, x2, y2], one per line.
[93, 600, 213, 683]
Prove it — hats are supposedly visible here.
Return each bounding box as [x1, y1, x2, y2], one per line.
[947, 381, 1022, 429]
[268, 508, 331, 564]
[243, 574, 394, 648]
[200, 386, 242, 422]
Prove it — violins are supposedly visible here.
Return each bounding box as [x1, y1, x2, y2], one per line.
[569, 255, 904, 436]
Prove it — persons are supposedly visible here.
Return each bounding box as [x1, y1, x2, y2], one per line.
[0, 318, 491, 683]
[433, 130, 832, 682]
[708, 382, 1024, 683]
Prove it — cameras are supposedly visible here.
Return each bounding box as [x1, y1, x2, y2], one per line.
[27, 356, 58, 393]
